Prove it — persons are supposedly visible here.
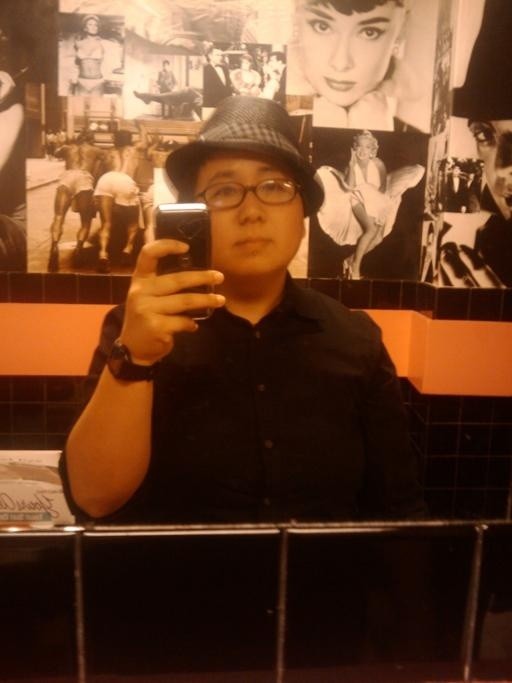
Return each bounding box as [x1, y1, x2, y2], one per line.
[288, 1, 426, 133]
[0, 15, 286, 272]
[312, 128, 426, 280]
[62, 93, 434, 526]
[424, 0, 512, 291]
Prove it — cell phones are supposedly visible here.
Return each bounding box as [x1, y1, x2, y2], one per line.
[153, 203, 212, 320]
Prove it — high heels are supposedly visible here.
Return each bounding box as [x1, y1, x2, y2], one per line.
[343, 259, 364, 281]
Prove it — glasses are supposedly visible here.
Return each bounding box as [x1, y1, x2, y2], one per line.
[193, 177, 301, 209]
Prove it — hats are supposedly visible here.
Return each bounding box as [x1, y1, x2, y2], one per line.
[165, 95, 324, 215]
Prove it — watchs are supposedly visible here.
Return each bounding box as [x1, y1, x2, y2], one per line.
[103, 341, 160, 383]
